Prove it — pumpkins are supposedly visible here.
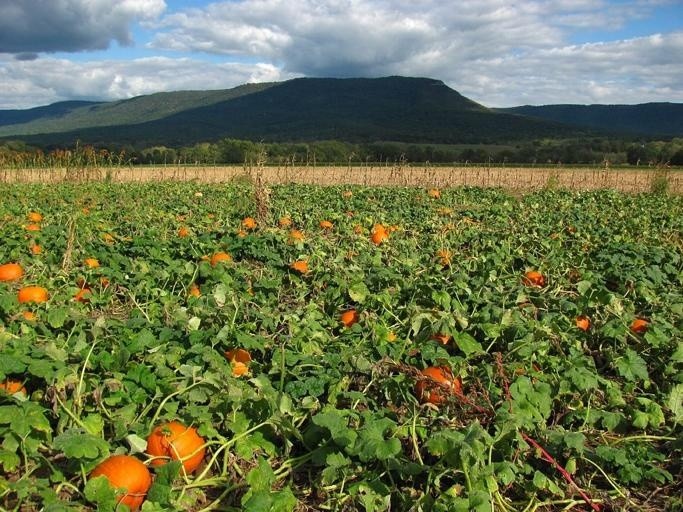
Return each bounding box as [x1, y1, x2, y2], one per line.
[0, 186, 682, 512]
